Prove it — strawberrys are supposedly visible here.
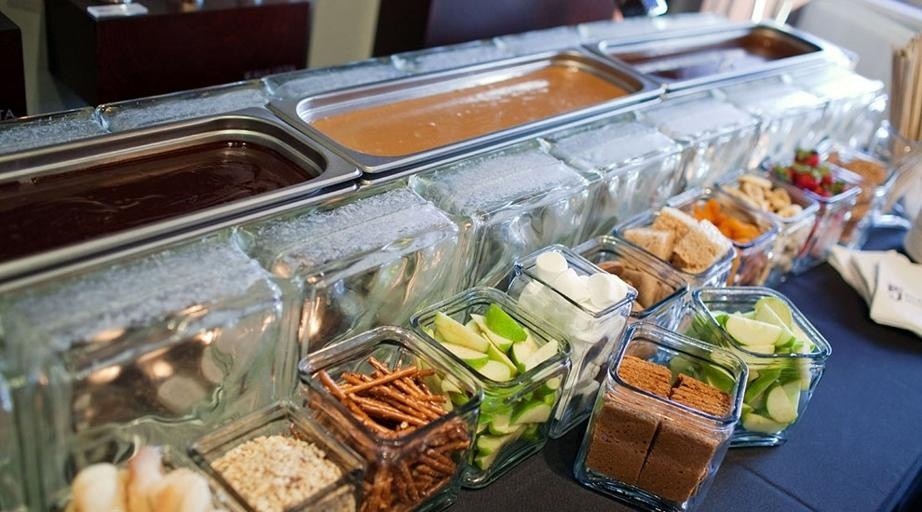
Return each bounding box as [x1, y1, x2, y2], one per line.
[770, 148, 852, 259]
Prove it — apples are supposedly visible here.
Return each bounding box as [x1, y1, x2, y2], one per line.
[669, 298, 817, 434]
[410, 302, 564, 471]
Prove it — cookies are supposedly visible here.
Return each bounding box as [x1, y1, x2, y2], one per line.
[584, 352, 734, 502]
[289, 354, 469, 512]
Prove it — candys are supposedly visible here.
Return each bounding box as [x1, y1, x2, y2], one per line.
[518, 250, 628, 421]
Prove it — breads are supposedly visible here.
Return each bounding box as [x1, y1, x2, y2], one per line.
[623, 207, 733, 307]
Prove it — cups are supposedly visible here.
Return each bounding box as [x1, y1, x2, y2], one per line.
[502, 241, 639, 440]
[817, 140, 901, 249]
[192, 402, 366, 511]
[296, 325, 482, 510]
[760, 153, 862, 275]
[49, 444, 248, 511]
[672, 286, 831, 448]
[565, 235, 687, 366]
[847, 120, 919, 213]
[572, 320, 752, 510]
[713, 167, 821, 283]
[613, 206, 737, 333]
[666, 184, 784, 285]
[407, 285, 574, 489]
